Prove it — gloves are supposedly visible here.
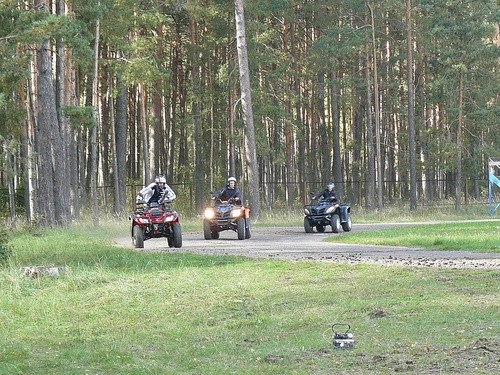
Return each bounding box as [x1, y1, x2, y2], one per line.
[312, 196, 316, 200]
[235, 197, 238, 202]
[330, 196, 334, 201]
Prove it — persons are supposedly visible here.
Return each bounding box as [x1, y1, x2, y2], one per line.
[310, 182, 341, 206]
[211, 176, 241, 207]
[135, 174, 176, 208]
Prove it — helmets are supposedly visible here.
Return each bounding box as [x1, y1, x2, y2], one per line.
[327, 181, 334, 188]
[227, 177, 237, 190]
[156, 174, 166, 185]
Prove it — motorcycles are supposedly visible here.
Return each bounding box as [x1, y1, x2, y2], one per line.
[303, 191, 352, 233]
[203, 191, 251, 239]
[129, 190, 182, 248]
